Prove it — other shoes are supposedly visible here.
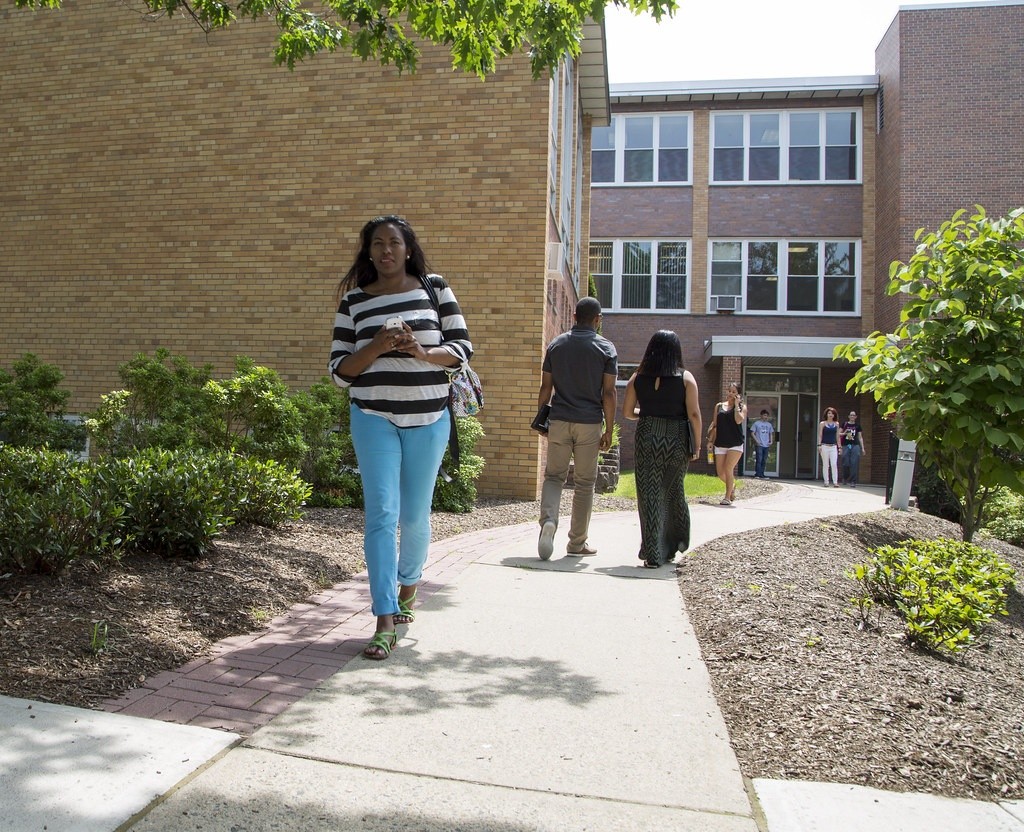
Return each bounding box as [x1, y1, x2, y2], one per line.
[824, 484, 829, 487]
[755, 475, 760, 479]
[567, 542, 597, 557]
[539, 521, 556, 560]
[643, 560, 657, 569]
[834, 484, 839, 487]
[668, 557, 675, 561]
[760, 475, 770, 480]
[850, 482, 855, 487]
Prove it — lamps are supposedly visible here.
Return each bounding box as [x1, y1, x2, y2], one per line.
[892, 439, 916, 510]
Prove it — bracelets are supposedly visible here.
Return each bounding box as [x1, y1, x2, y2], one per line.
[734, 407, 741, 412]
[709, 441, 713, 442]
[838, 447, 842, 449]
[817, 445, 821, 446]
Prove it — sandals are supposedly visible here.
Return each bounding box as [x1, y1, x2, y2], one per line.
[394, 584, 417, 625]
[364, 628, 397, 661]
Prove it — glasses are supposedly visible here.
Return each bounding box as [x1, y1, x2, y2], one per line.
[372, 214, 406, 226]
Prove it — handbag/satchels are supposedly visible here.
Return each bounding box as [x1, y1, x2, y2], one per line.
[416, 273, 485, 417]
[841, 422, 847, 445]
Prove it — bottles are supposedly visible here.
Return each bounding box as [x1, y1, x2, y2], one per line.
[707, 448, 714, 464]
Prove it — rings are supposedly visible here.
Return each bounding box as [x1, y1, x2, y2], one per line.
[391, 342, 394, 347]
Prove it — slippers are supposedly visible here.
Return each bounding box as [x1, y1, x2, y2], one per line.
[730, 483, 736, 499]
[719, 498, 732, 505]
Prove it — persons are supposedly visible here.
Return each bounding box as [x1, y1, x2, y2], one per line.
[839, 410, 866, 487]
[706, 383, 747, 505]
[622, 330, 702, 568]
[750, 410, 774, 479]
[818, 407, 841, 488]
[327, 214, 474, 659]
[538, 297, 618, 560]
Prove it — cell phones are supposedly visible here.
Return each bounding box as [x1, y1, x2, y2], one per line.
[740, 394, 743, 399]
[386, 316, 404, 339]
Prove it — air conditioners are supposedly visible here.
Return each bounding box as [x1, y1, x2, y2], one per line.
[716, 295, 737, 312]
[547, 242, 567, 281]
